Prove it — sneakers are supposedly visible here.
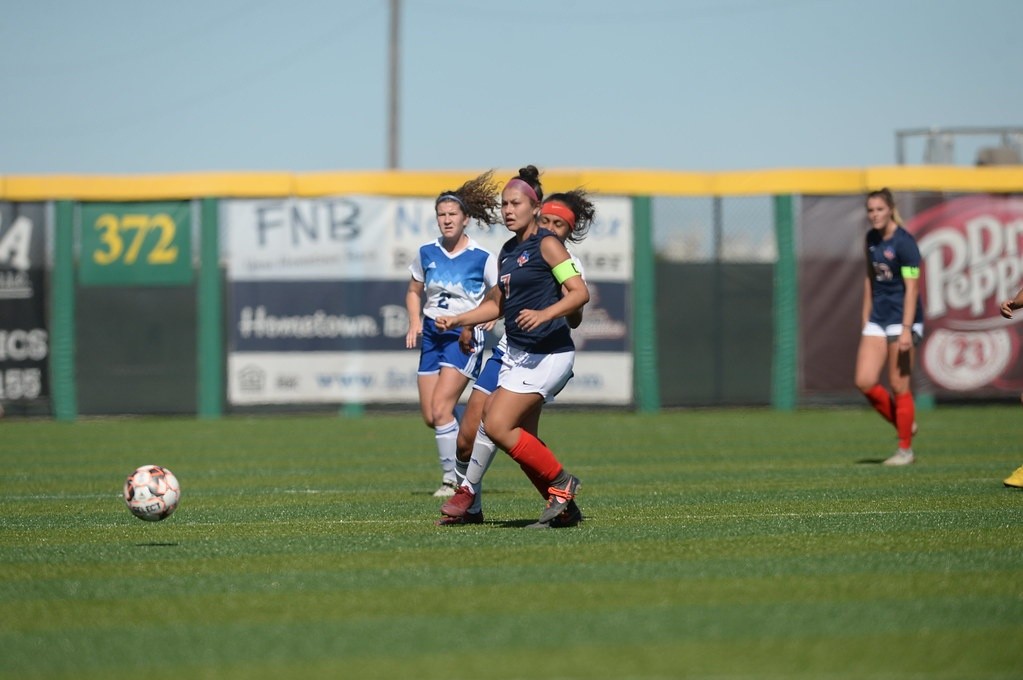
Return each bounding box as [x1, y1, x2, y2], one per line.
[1004, 465, 1023, 487]
[911, 422, 918, 435]
[440, 485, 476, 518]
[539, 474, 581, 524]
[526, 505, 580, 529]
[433, 484, 454, 497]
[882, 448, 915, 465]
[436, 510, 483, 526]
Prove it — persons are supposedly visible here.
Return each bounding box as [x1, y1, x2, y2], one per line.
[435, 163, 589, 523]
[405, 170, 500, 497]
[1000, 288, 1023, 488]
[855, 187, 925, 466]
[432, 190, 595, 527]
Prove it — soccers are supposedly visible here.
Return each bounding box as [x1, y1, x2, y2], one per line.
[123, 464, 182, 522]
